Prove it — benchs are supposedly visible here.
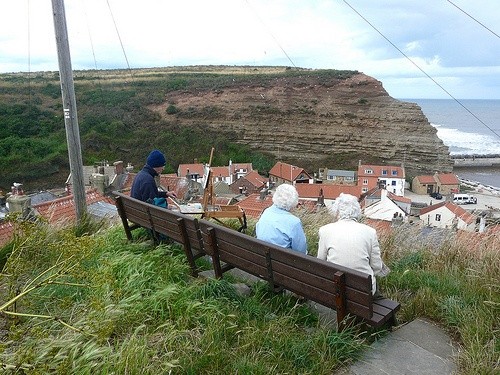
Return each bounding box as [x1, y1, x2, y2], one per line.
[112, 191, 204, 274]
[197, 216, 400, 342]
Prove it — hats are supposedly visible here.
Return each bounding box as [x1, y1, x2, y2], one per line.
[146, 150, 166, 167]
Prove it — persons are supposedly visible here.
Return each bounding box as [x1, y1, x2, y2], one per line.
[316, 191, 384, 297]
[254, 183, 310, 299]
[130, 150, 178, 243]
